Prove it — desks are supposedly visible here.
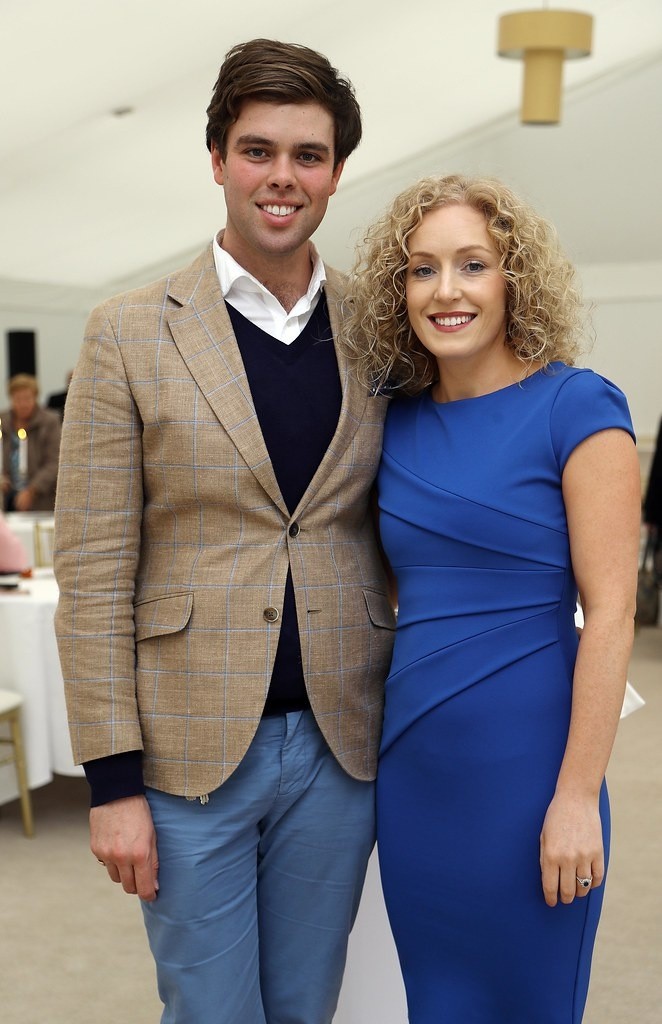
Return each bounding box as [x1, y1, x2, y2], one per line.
[0, 568, 86, 803]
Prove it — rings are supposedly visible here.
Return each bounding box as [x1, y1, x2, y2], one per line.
[577, 875, 593, 889]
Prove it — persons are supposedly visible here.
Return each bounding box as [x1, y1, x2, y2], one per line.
[1, 368, 75, 515]
[51, 37, 409, 1022]
[331, 171, 642, 1024]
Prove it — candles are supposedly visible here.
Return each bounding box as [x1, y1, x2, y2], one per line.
[18, 430, 29, 484]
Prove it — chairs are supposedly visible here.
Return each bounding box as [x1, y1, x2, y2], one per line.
[1, 689, 37, 838]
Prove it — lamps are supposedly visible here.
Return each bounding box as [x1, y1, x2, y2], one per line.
[497, 7, 591, 127]
